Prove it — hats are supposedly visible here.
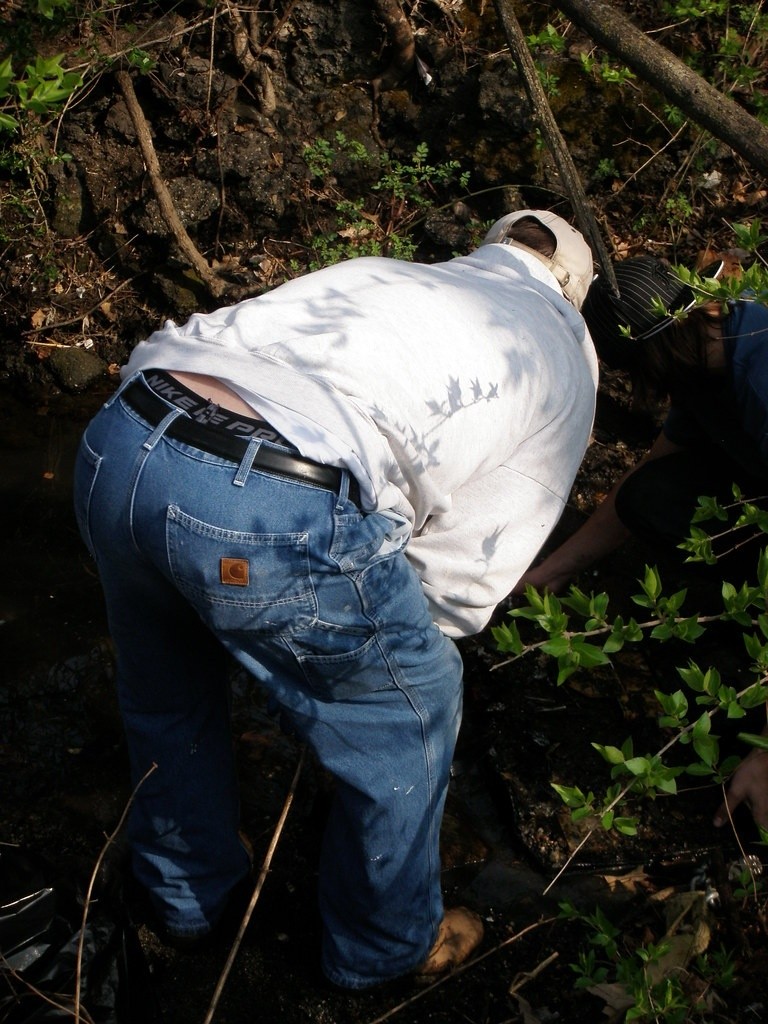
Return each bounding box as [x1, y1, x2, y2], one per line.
[479, 209, 594, 312]
[582, 256, 724, 365]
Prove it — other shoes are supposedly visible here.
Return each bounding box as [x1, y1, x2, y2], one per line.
[408, 905, 484, 985]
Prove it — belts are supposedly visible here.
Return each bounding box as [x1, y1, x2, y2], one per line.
[119, 378, 362, 508]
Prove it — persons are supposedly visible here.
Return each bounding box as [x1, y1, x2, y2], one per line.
[510, 254, 768, 851]
[70, 209, 600, 991]
